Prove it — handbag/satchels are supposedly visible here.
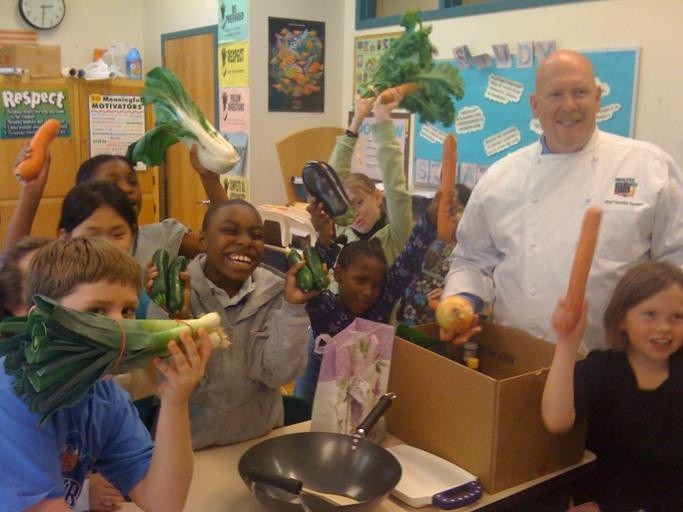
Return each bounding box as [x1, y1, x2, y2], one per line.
[312, 316, 396, 443]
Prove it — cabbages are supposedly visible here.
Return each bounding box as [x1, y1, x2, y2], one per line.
[125, 67, 242, 174]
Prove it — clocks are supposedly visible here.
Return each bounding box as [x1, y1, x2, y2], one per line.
[19, 0, 66, 30]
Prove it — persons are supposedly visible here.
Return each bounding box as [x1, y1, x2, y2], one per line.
[291, 83, 415, 404]
[1, 231, 213, 511]
[398, 179, 475, 322]
[436, 44, 682, 363]
[71, 142, 232, 298]
[537, 255, 682, 511]
[1, 138, 176, 324]
[0, 234, 60, 321]
[275, 186, 466, 428]
[136, 194, 312, 455]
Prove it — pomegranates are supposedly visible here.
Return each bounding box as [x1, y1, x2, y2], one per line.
[435, 295, 474, 334]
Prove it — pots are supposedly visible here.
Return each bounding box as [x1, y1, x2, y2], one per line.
[239, 392, 402, 512]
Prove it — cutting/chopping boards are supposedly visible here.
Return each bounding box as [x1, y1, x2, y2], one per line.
[375, 444, 484, 509]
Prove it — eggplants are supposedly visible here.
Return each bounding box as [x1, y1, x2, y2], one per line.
[302, 161, 356, 226]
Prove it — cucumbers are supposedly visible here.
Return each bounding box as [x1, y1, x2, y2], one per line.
[167, 256, 187, 313]
[302, 244, 331, 291]
[287, 249, 313, 293]
[150, 248, 169, 304]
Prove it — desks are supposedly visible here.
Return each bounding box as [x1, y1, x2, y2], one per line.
[118, 417, 597, 512]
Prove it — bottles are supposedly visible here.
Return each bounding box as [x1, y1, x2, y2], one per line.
[125, 45, 142, 79]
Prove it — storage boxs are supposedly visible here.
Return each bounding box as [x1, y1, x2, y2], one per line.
[384, 322, 585, 495]
[0, 43, 62, 79]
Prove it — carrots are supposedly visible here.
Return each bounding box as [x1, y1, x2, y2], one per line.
[436, 134, 457, 242]
[379, 82, 417, 104]
[553, 206, 603, 337]
[13, 118, 60, 182]
